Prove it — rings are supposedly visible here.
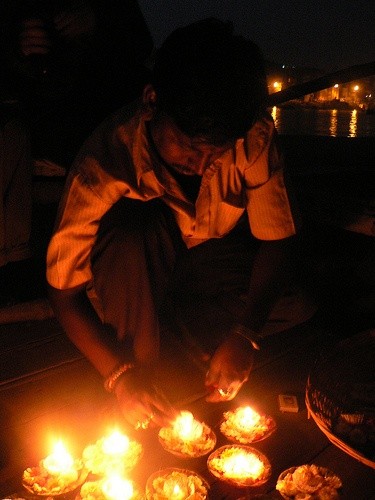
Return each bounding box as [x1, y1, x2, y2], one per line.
[216, 386, 230, 397]
[142, 417, 151, 429]
[135, 421, 142, 430]
[148, 409, 158, 419]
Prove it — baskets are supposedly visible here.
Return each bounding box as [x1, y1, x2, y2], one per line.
[304, 329, 375, 471]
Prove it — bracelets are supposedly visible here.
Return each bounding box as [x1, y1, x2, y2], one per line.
[104, 362, 131, 388]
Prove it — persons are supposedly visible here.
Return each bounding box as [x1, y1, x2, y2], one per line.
[43, 14, 302, 415]
[0, 0, 155, 329]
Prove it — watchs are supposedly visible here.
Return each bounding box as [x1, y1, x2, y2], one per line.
[231, 323, 266, 350]
